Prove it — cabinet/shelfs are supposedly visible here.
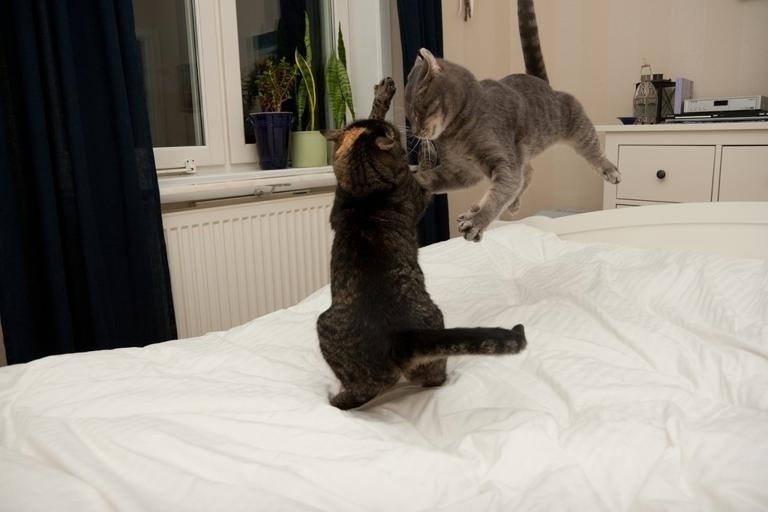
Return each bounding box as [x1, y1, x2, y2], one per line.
[590, 118, 767, 215]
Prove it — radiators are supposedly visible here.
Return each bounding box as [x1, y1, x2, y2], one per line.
[154, 191, 343, 342]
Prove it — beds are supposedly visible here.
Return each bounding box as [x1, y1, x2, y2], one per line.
[1, 196, 768, 512]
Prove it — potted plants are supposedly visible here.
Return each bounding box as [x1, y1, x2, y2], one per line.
[237, 8, 330, 171]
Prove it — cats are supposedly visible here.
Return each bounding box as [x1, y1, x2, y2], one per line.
[391, 48, 621, 243]
[316, 76, 527, 411]
